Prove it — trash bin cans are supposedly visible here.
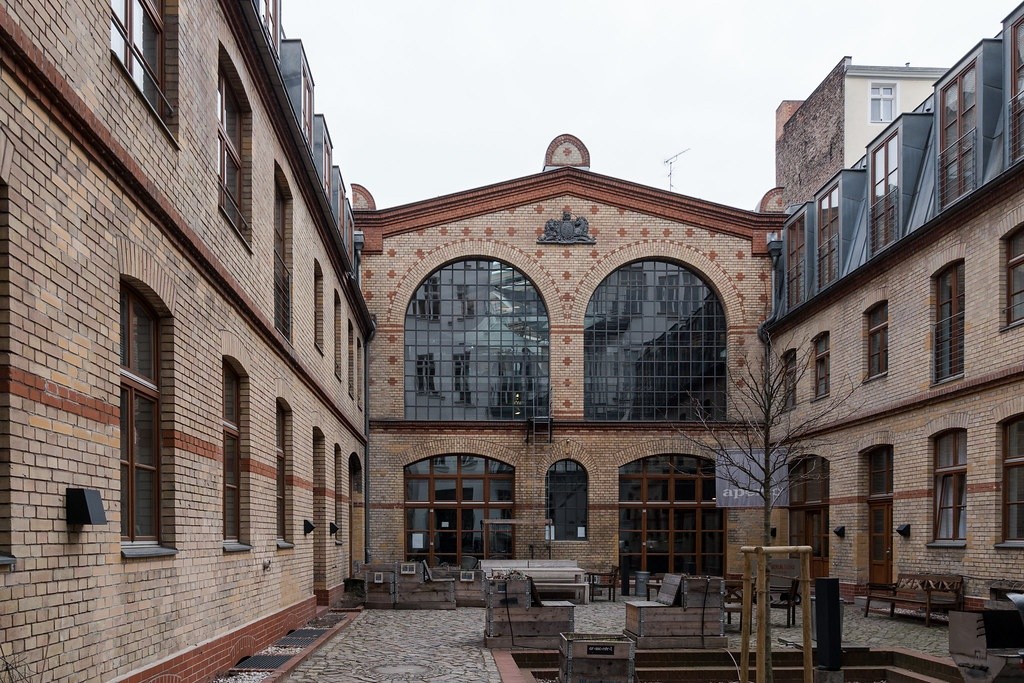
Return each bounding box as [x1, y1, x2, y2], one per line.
[635, 571, 651, 596]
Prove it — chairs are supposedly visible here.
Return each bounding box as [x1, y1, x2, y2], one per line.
[723, 579, 744, 629]
[769, 576, 800, 629]
[645, 573, 666, 601]
[585, 566, 617, 602]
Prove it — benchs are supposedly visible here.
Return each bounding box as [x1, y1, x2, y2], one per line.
[534, 582, 589, 604]
[395, 559, 457, 610]
[623, 574, 728, 650]
[864, 573, 964, 628]
[484, 573, 576, 650]
